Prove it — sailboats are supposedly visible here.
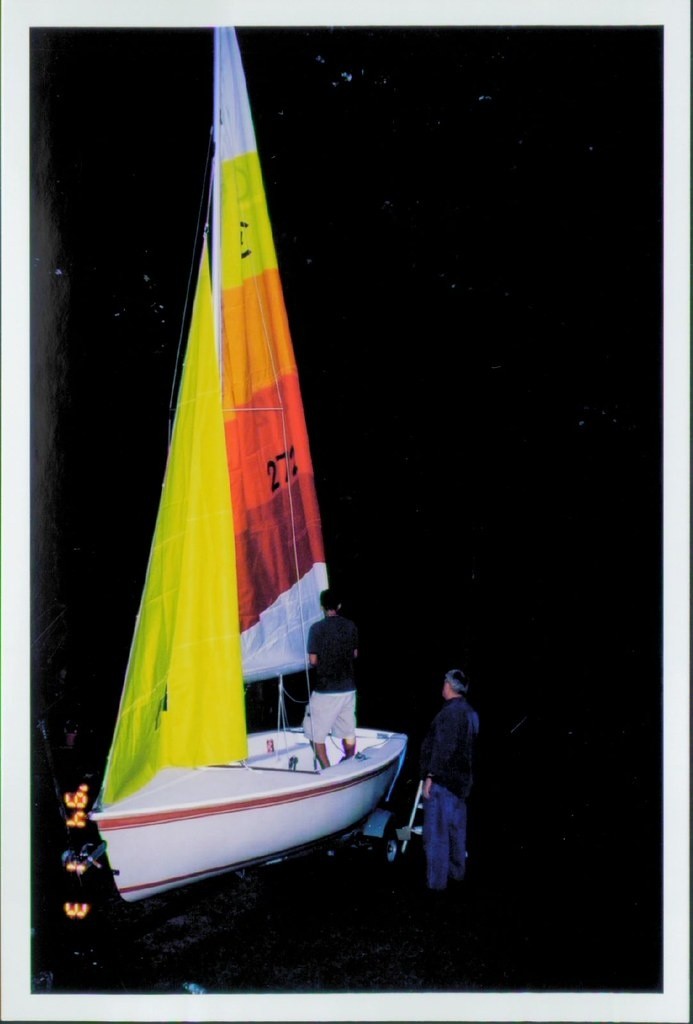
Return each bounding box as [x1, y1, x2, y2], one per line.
[76, 26, 411, 907]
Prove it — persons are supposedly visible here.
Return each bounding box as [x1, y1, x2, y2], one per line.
[303, 591, 358, 769]
[423, 671, 480, 889]
